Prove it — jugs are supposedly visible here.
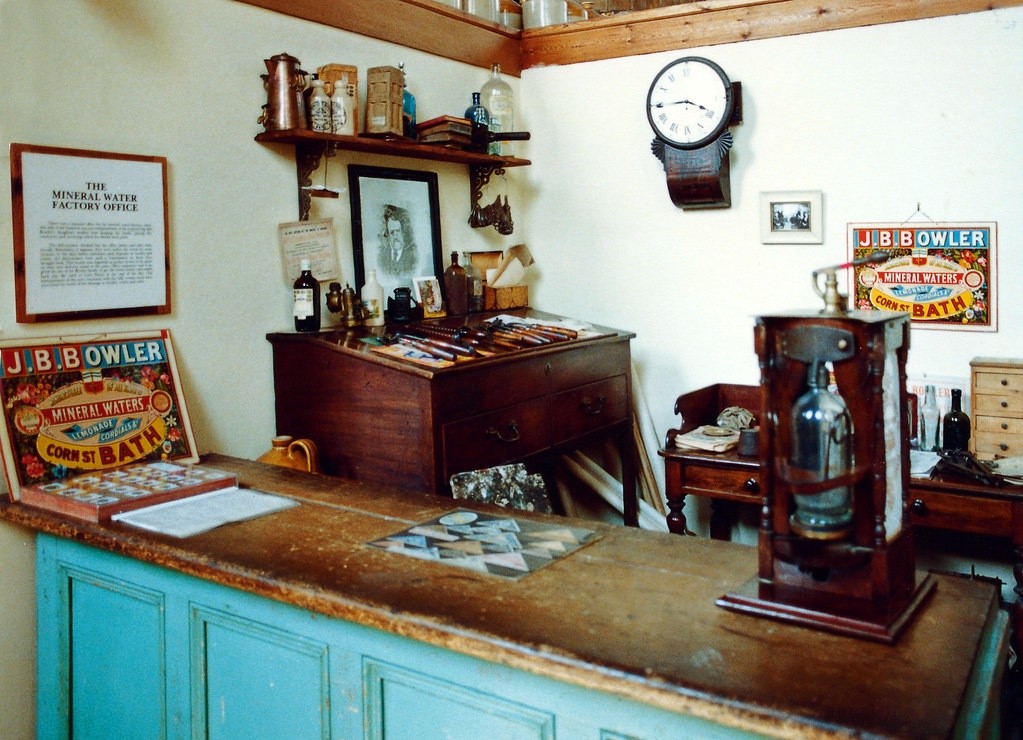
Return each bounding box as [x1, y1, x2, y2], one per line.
[264, 53, 310, 129]
[257, 436, 319, 473]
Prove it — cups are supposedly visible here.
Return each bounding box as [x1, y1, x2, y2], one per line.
[737, 427, 759, 456]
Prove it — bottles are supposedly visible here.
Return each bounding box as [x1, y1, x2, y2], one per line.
[792, 357, 851, 525]
[461, 252, 482, 312]
[303, 73, 319, 129]
[443, 250, 467, 316]
[361, 270, 385, 326]
[943, 388, 971, 458]
[330, 80, 354, 136]
[397, 62, 416, 139]
[294, 260, 321, 332]
[921, 384, 940, 452]
[308, 80, 331, 134]
[480, 63, 514, 157]
[465, 93, 488, 154]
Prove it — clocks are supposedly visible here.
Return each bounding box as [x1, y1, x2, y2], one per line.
[646, 56, 744, 212]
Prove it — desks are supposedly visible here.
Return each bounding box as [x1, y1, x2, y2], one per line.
[656, 383, 1023, 606]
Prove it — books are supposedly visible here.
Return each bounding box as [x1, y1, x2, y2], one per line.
[910, 449, 948, 480]
[674, 425, 740, 453]
[416, 115, 473, 150]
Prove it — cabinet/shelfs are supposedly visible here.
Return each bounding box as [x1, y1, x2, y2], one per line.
[34, 533, 776, 740]
[969, 356, 1023, 461]
[264, 307, 641, 528]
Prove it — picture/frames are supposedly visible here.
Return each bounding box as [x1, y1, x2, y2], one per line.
[759, 191, 824, 245]
[347, 163, 446, 321]
